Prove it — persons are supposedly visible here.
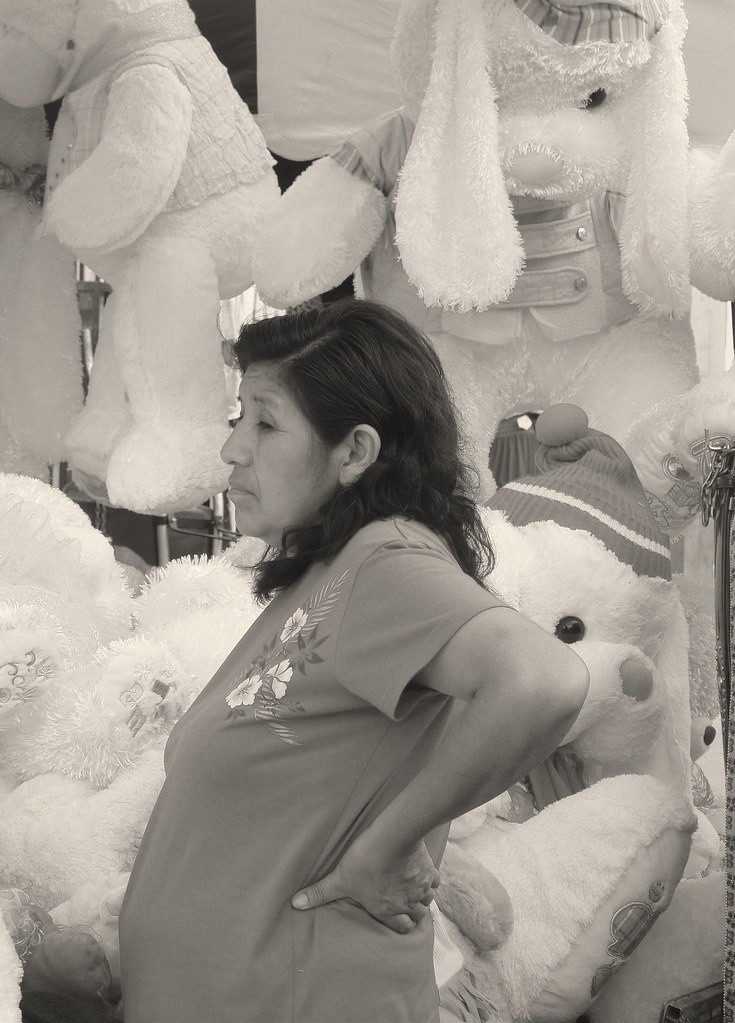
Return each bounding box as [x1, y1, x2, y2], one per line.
[118, 300, 590, 1023]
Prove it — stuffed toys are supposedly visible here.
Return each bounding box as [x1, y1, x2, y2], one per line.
[0, 0, 734, 541]
[0, 402, 726, 1023]
